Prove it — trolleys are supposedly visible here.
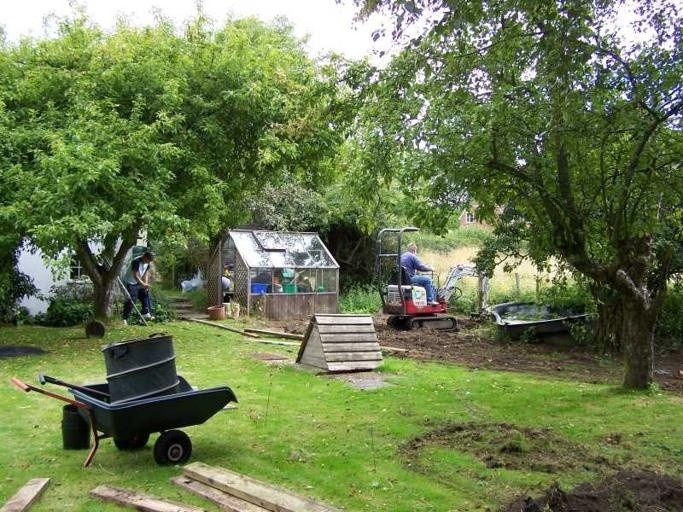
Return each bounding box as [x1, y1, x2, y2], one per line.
[10, 374, 240, 466]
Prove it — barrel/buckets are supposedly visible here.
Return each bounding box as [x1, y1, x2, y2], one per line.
[61, 404, 91, 450]
[101, 334, 180, 405]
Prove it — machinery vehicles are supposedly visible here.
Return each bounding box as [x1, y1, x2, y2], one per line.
[375, 226, 489, 332]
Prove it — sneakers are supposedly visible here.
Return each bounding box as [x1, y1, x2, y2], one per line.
[122, 319, 127, 325]
[427, 299, 438, 305]
[142, 313, 151, 319]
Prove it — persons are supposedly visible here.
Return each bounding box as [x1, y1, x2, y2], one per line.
[121, 252, 154, 325]
[394, 242, 440, 305]
